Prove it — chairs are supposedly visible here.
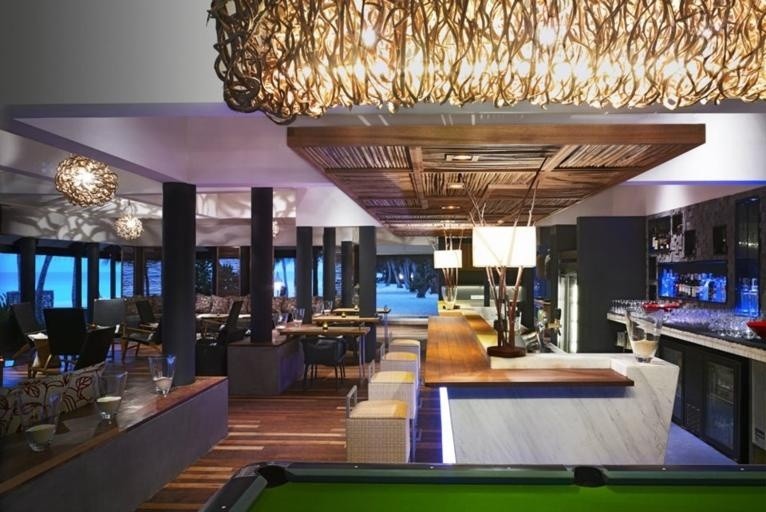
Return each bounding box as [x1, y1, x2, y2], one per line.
[367, 358, 424, 442]
[0, 302, 68, 367]
[136, 299, 156, 325]
[379, 342, 424, 411]
[301, 336, 348, 392]
[42, 307, 90, 371]
[39, 325, 117, 377]
[387, 332, 422, 387]
[345, 385, 412, 463]
[93, 297, 125, 360]
[121, 315, 165, 361]
[197, 299, 242, 377]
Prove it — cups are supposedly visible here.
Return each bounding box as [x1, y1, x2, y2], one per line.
[624, 305, 664, 364]
[93, 365, 128, 423]
[289, 308, 307, 327]
[441, 285, 458, 312]
[147, 352, 178, 397]
[271, 312, 290, 334]
[322, 300, 334, 316]
[16, 385, 65, 453]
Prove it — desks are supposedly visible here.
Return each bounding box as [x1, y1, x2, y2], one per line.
[325, 305, 392, 353]
[313, 314, 381, 362]
[275, 323, 371, 384]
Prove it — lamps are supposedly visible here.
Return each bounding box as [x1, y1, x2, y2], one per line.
[54, 155, 119, 208]
[433, 248, 462, 310]
[471, 225, 538, 359]
[206, 0, 766, 123]
[116, 198, 144, 241]
